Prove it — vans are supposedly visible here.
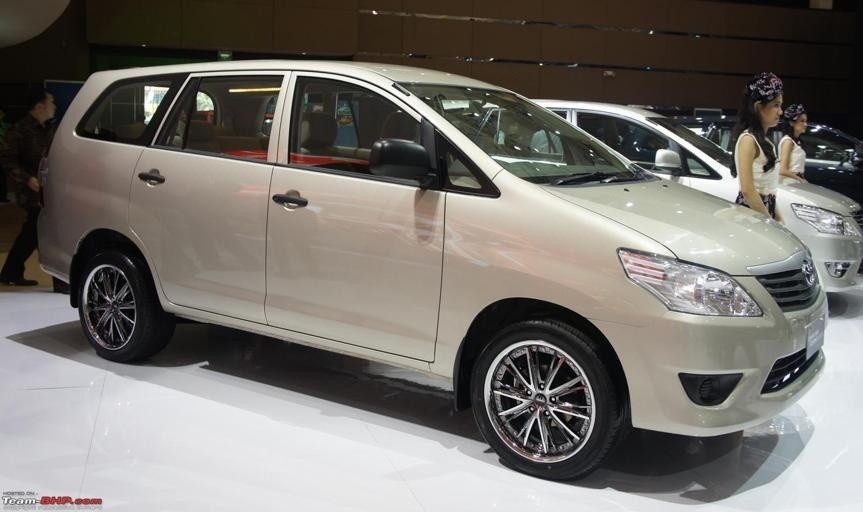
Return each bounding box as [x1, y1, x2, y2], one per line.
[26, 55, 828, 491]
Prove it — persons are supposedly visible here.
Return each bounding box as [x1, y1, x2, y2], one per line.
[0, 88, 57, 286]
[730, 72, 784, 222]
[778, 104, 808, 184]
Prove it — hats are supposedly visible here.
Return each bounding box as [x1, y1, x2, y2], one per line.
[749, 72, 784, 103]
[784, 104, 807, 120]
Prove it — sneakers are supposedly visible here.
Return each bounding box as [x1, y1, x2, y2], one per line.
[0, 274, 40, 289]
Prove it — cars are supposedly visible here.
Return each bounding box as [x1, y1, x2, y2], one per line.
[438, 93, 862, 294]
[648, 103, 862, 212]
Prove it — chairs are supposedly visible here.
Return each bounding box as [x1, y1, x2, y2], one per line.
[173, 119, 223, 153]
[300, 112, 344, 156]
